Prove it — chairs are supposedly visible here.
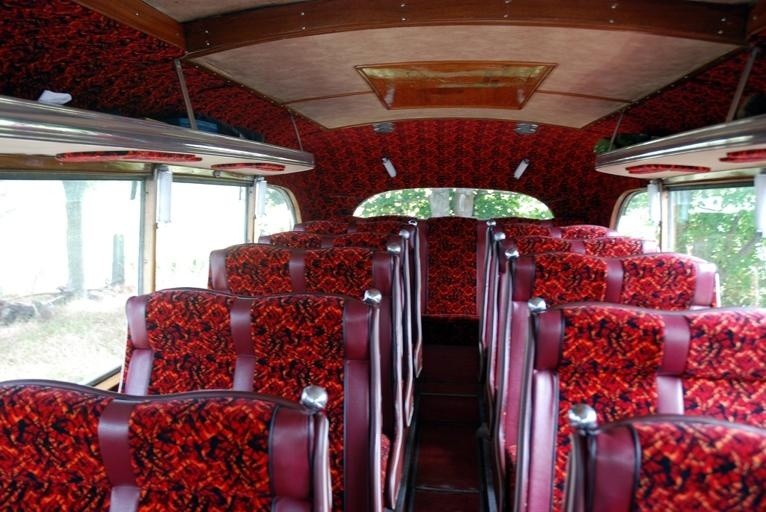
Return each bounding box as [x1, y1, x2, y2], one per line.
[421, 217, 484, 322]
